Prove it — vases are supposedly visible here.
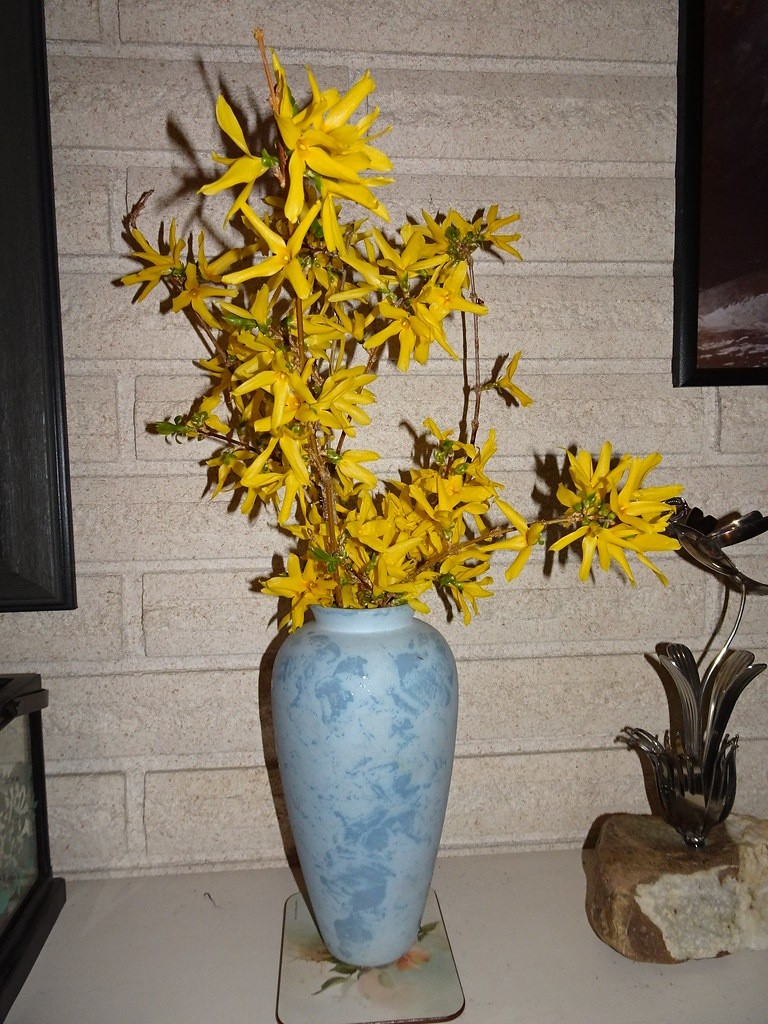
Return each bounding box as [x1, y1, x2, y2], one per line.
[268, 599, 458, 968]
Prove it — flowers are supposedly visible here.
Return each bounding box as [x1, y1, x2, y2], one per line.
[110, 26, 687, 637]
